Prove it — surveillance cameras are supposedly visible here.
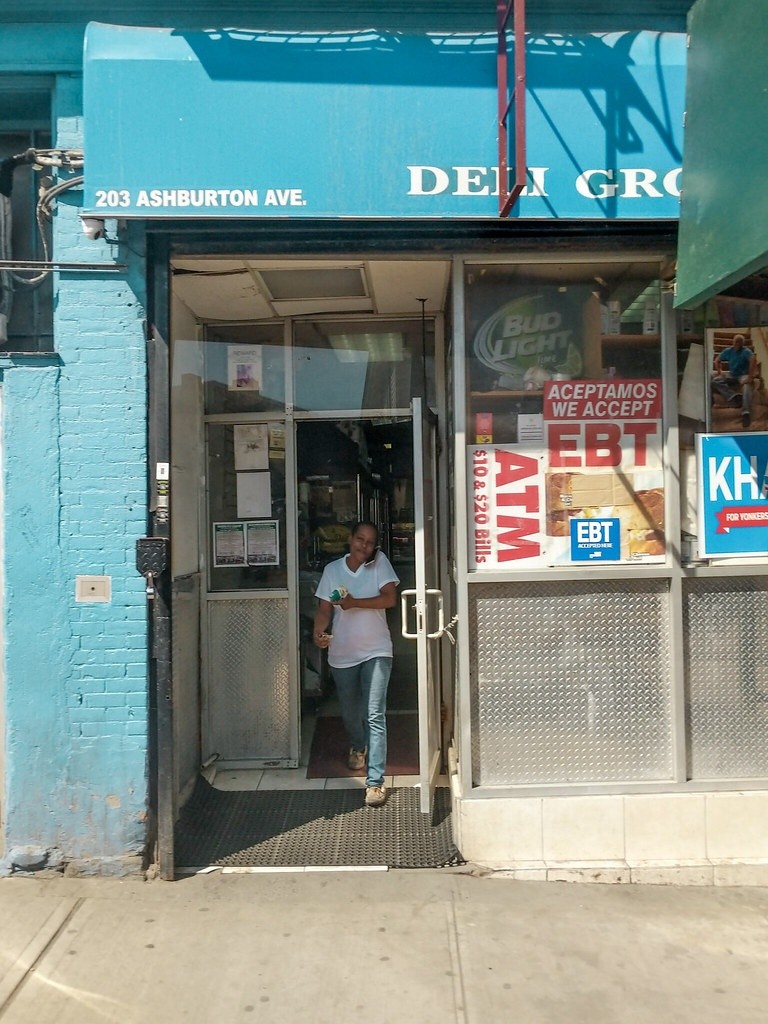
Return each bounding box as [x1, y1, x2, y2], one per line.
[81, 219, 104, 240]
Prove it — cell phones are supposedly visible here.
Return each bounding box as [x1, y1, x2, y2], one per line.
[363, 547, 381, 567]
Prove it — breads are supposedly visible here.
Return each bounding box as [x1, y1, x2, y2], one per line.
[545, 472, 667, 554]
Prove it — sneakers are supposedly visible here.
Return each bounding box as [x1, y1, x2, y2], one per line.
[348, 744, 367, 770]
[364, 783, 387, 805]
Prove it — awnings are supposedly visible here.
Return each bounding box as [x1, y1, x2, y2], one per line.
[673, 0, 768, 311]
[80, 12, 687, 218]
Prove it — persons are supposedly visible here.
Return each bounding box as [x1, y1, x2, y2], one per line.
[314, 521, 400, 805]
[711, 335, 756, 428]
